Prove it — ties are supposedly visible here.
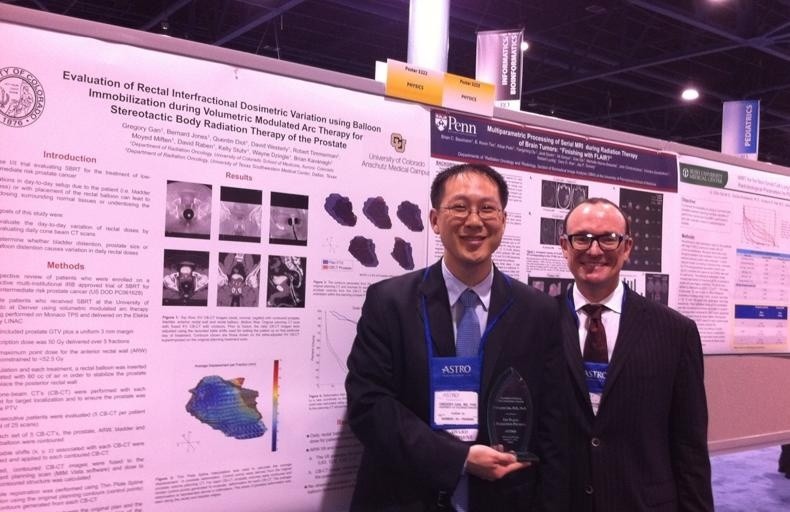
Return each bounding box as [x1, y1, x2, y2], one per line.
[582, 304, 608, 364]
[450, 288, 481, 511]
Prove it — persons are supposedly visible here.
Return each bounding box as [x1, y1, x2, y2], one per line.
[548, 196, 716, 512]
[343, 162, 595, 512]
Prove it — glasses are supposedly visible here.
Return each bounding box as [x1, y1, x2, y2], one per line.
[563, 231, 626, 252]
[438, 201, 503, 220]
